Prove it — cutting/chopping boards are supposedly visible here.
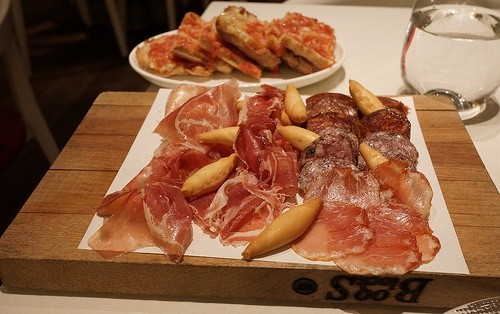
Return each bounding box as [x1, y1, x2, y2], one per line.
[1, 91, 500, 309]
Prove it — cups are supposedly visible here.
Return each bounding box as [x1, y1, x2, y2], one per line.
[399, 0, 500, 119]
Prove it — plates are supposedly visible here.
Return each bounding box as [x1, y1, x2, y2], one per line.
[129, 29, 345, 92]
